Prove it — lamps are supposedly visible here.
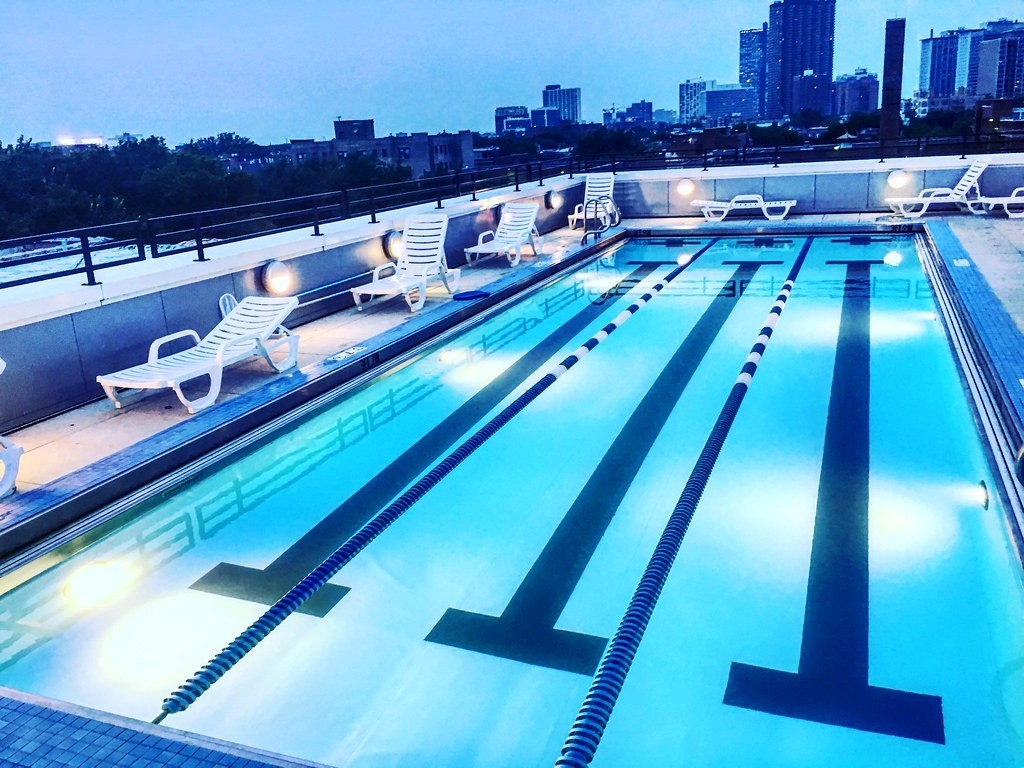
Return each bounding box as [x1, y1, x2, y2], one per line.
[384, 232, 403, 259]
[261, 261, 291, 293]
[887, 168, 907, 188]
[548, 191, 561, 209]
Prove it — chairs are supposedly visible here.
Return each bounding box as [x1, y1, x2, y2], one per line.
[464, 202, 543, 268]
[691, 194, 797, 223]
[884, 159, 1024, 218]
[350, 214, 461, 313]
[96, 296, 300, 416]
[568, 172, 620, 230]
